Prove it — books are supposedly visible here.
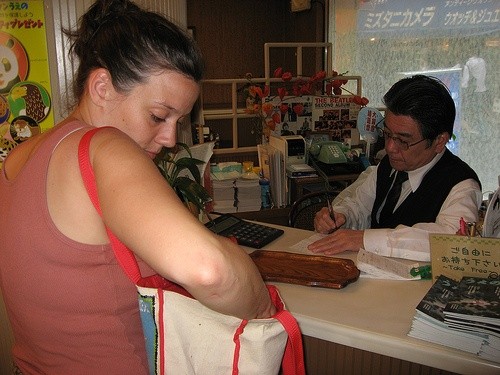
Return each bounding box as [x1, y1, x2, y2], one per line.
[406, 274, 499, 366]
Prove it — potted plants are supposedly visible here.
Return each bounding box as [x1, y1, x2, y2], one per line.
[153, 143, 217, 230]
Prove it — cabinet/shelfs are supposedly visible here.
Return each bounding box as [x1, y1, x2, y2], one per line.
[231, 174, 359, 227]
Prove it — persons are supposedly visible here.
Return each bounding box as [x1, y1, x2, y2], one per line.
[281, 110, 357, 149]
[0, 1, 277, 375]
[306, 75, 483, 262]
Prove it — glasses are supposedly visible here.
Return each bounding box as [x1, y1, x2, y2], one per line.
[375, 117, 427, 150]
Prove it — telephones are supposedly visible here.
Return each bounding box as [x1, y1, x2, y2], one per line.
[309, 140, 350, 165]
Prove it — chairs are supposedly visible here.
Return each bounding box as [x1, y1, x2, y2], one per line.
[289, 190, 341, 231]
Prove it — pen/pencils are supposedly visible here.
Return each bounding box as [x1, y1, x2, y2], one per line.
[325, 194, 337, 232]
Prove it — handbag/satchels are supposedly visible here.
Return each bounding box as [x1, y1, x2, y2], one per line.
[77, 126, 306, 375]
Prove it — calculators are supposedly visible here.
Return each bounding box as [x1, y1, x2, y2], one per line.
[201, 213, 285, 250]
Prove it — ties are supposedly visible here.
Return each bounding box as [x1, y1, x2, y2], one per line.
[379, 171, 408, 229]
